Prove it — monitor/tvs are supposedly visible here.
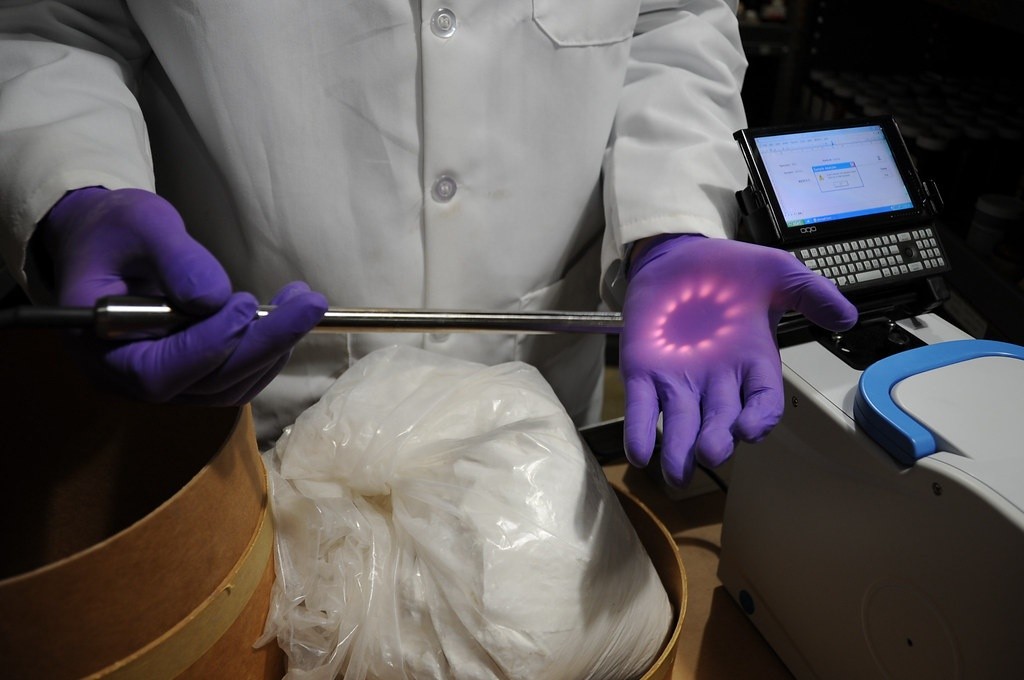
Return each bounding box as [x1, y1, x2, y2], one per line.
[734, 118, 939, 241]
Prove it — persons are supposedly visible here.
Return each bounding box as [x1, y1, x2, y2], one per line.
[0, 0, 859, 490]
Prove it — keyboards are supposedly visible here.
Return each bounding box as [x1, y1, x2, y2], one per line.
[786, 226, 951, 293]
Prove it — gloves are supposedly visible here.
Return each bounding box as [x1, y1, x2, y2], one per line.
[620, 233, 857, 489]
[27, 186, 328, 413]
